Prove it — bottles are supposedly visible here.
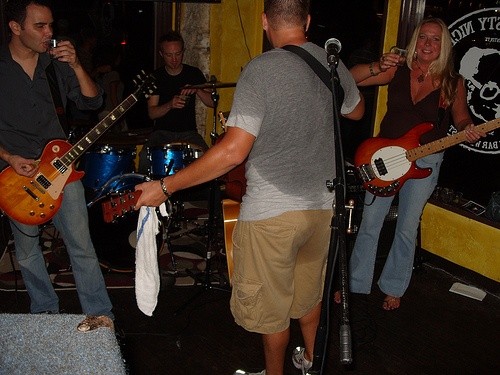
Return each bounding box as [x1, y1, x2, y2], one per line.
[485, 192, 500, 220]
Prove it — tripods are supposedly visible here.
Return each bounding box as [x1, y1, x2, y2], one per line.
[168, 90, 231, 320]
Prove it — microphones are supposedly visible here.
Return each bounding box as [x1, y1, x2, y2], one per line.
[325, 38, 342, 69]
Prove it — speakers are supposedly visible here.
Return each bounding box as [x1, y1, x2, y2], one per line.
[0, 312, 128, 375]
[347, 187, 422, 272]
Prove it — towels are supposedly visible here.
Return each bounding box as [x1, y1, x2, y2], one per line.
[135, 205, 161, 318]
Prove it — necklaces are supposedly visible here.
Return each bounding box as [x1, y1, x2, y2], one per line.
[413, 62, 429, 82]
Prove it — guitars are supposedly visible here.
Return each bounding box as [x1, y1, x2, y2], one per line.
[0, 68, 159, 225]
[101, 130, 248, 225]
[355, 117, 500, 198]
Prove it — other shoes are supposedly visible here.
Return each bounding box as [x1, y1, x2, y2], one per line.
[208, 211, 223, 233]
[292, 346, 313, 371]
[382, 294, 400, 310]
[231, 368, 267, 375]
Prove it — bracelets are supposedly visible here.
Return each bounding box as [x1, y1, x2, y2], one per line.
[160, 178, 172, 198]
[369, 62, 376, 76]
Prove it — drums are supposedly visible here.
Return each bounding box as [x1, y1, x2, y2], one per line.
[84, 174, 174, 272]
[80, 144, 136, 196]
[148, 141, 207, 207]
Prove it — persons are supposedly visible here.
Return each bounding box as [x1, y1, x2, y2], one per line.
[349, 18, 487, 313]
[0, 0, 129, 346]
[138, 31, 219, 170]
[134, 0, 365, 375]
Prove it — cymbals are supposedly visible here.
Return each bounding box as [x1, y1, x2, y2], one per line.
[180, 81, 237, 89]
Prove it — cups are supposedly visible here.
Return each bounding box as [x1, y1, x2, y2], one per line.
[392, 48, 408, 66]
[433, 185, 463, 206]
[178, 95, 188, 105]
[47, 39, 61, 59]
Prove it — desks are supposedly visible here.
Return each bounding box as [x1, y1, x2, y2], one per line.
[75, 133, 146, 175]
